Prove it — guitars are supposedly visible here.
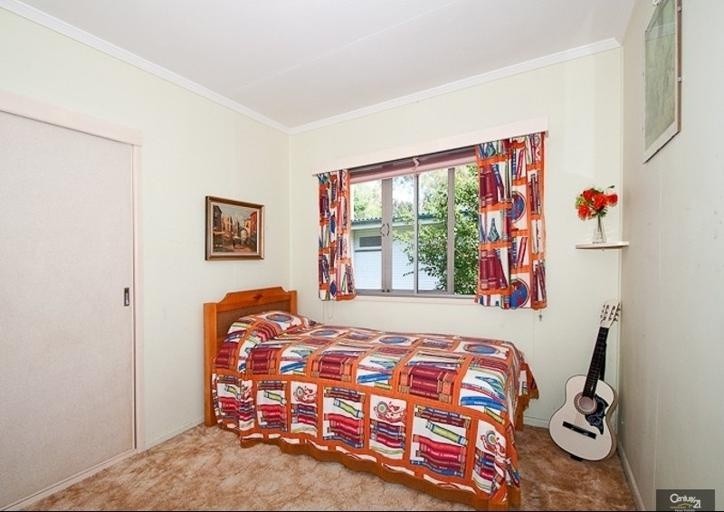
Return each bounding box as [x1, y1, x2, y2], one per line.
[548, 299, 621, 462]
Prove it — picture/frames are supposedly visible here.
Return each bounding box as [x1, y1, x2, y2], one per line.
[203, 194, 266, 264]
[641, 0, 681, 166]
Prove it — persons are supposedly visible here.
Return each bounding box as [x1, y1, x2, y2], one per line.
[233, 239, 236, 249]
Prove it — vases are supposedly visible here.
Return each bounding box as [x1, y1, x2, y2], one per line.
[591, 214, 607, 243]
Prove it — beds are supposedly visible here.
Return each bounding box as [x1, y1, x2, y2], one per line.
[200, 284, 541, 511]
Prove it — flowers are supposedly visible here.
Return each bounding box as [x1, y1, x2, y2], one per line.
[574, 180, 619, 244]
